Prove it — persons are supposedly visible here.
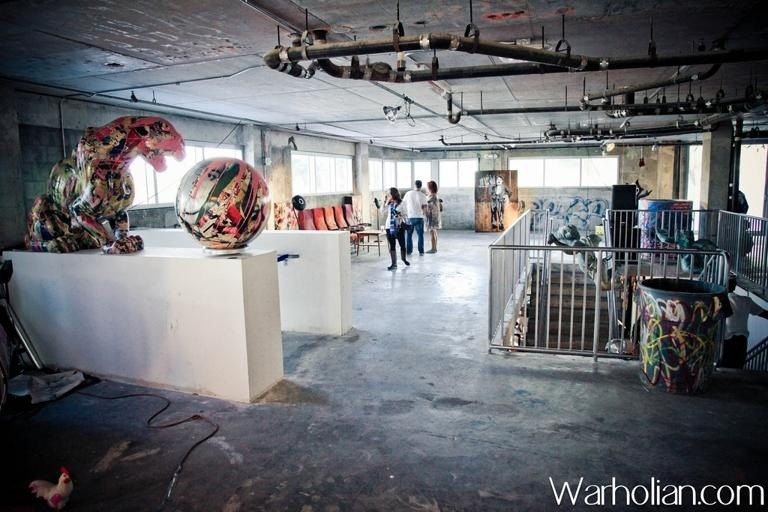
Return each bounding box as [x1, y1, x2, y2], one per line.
[488, 177, 514, 232]
[722, 271, 767, 369]
[380, 187, 410, 269]
[404, 179, 427, 256]
[653, 222, 726, 278]
[425, 181, 443, 253]
[729, 180, 749, 257]
[548, 225, 612, 290]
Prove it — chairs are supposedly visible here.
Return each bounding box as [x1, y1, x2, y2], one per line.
[297, 204, 371, 253]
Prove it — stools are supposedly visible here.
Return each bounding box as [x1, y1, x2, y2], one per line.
[356, 230, 382, 256]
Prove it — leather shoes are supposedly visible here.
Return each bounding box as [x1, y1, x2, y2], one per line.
[426, 249, 434, 253]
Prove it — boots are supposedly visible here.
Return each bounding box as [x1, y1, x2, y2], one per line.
[401, 247, 410, 266]
[387, 250, 397, 269]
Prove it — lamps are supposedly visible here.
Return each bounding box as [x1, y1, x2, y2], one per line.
[382, 95, 416, 128]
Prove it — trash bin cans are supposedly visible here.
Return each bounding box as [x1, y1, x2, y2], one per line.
[637, 278, 733, 396]
